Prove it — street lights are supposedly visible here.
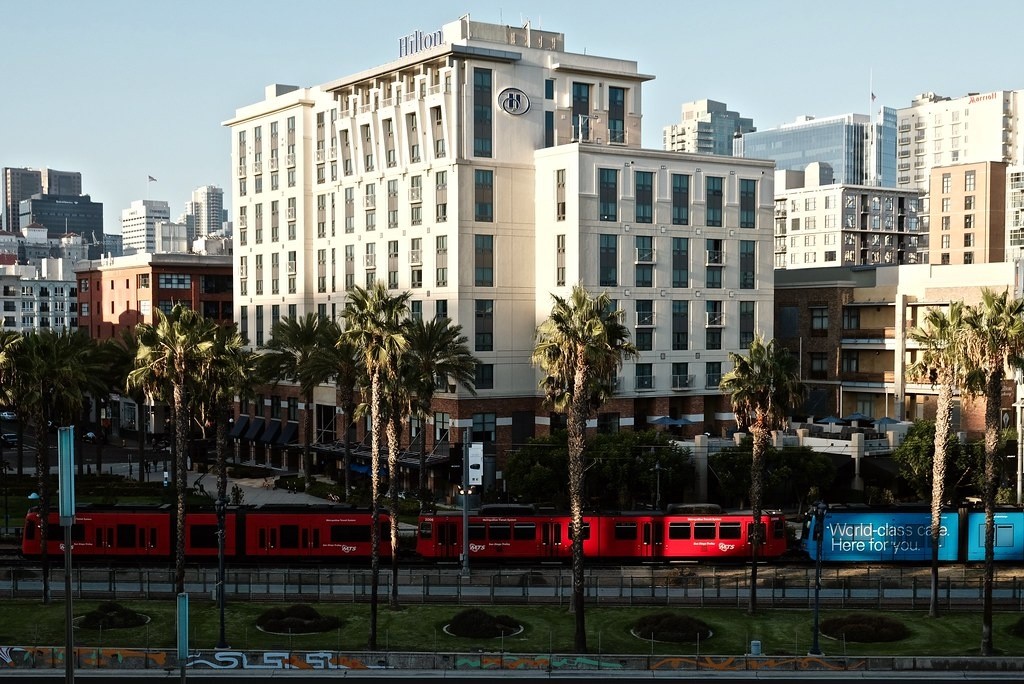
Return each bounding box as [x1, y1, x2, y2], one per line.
[653, 463, 661, 510]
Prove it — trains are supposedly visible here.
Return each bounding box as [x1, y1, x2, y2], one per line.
[18, 502, 1024, 568]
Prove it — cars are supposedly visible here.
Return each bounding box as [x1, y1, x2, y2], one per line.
[1, 434, 18, 446]
[42, 420, 61, 432]
[81, 432, 107, 444]
[0, 411, 17, 421]
